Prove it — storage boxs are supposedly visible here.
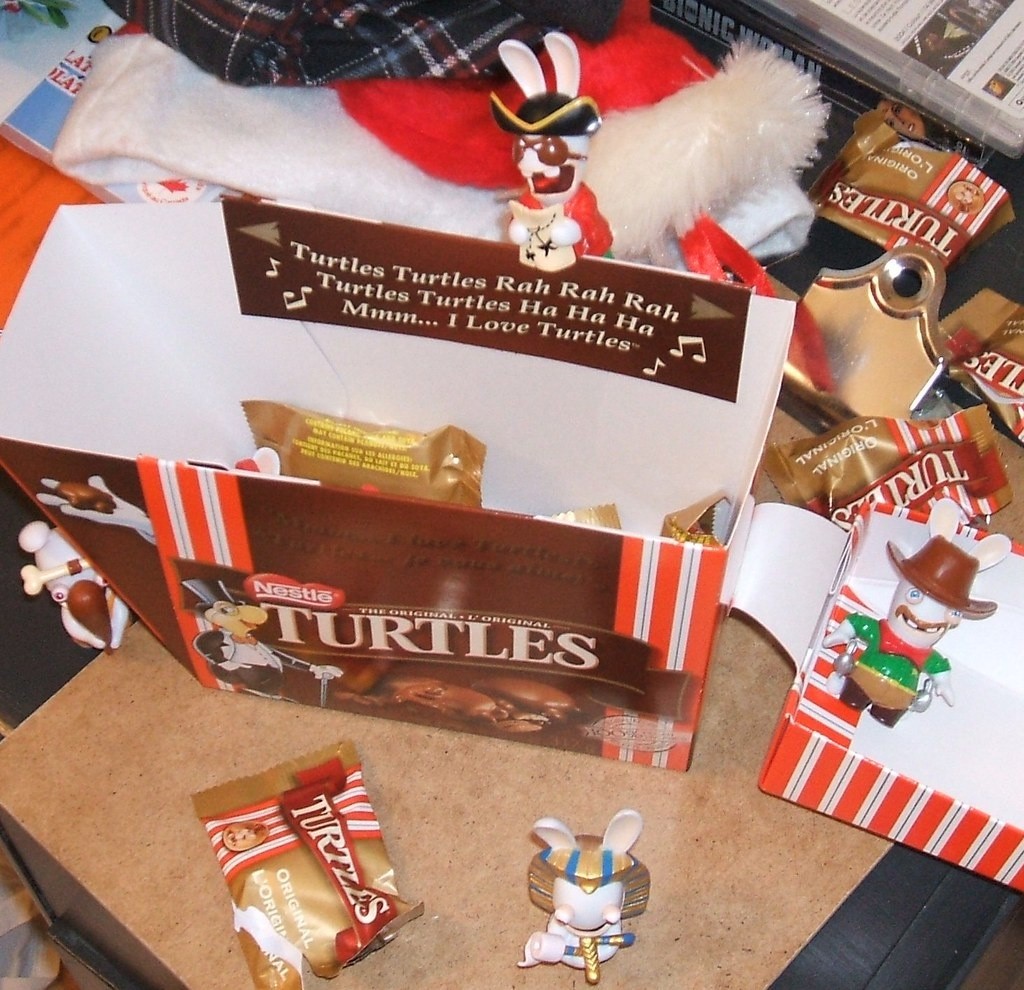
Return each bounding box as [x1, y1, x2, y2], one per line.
[757, 500, 1023, 894]
[0, 206, 796, 775]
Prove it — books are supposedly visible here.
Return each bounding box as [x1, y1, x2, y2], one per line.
[0, 0, 227, 204]
[655, 0, 1023, 169]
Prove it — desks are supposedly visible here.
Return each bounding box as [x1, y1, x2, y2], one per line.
[0, 465, 1023, 990]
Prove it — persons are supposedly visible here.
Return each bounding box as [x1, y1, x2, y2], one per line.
[866, 91, 925, 143]
